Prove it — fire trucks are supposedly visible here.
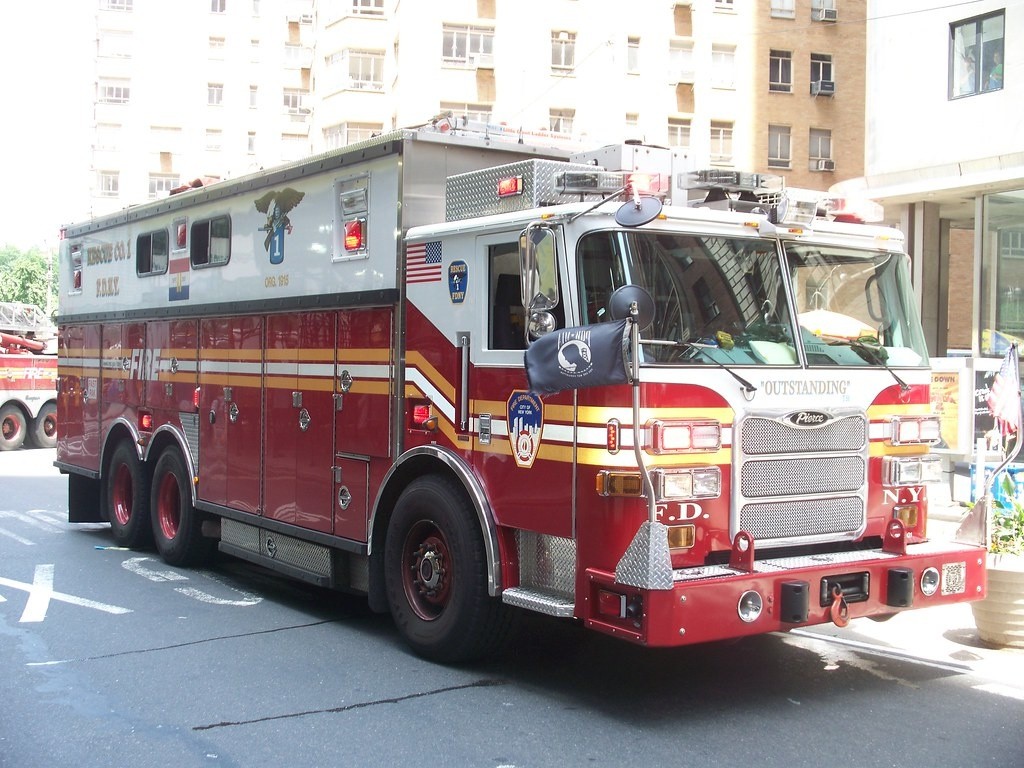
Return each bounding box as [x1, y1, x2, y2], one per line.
[52, 120, 987, 667]
[0, 302, 62, 450]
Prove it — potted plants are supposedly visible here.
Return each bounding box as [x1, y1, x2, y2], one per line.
[971, 473, 1024, 647]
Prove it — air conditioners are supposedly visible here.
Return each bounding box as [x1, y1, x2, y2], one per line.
[820, 9, 837, 22]
[812, 81, 835, 96]
[819, 160, 835, 172]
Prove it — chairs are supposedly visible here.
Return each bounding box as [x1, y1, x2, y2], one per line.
[494, 274, 565, 350]
